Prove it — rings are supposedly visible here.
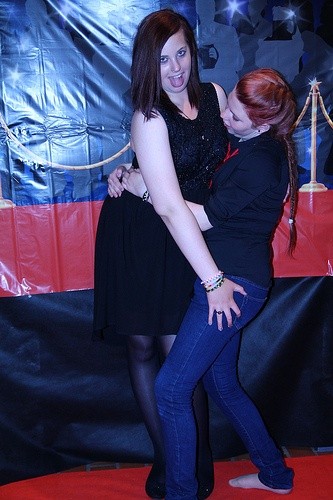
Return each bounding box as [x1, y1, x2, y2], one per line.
[216, 311, 223, 314]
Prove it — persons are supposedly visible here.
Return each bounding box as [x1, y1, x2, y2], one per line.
[104, 66, 299, 500]
[90, 9, 249, 500]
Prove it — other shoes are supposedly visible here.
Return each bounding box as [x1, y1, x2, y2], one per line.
[195, 455, 215, 500]
[145, 464, 167, 499]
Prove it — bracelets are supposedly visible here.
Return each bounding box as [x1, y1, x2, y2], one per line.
[142, 190, 151, 202]
[201, 273, 226, 294]
